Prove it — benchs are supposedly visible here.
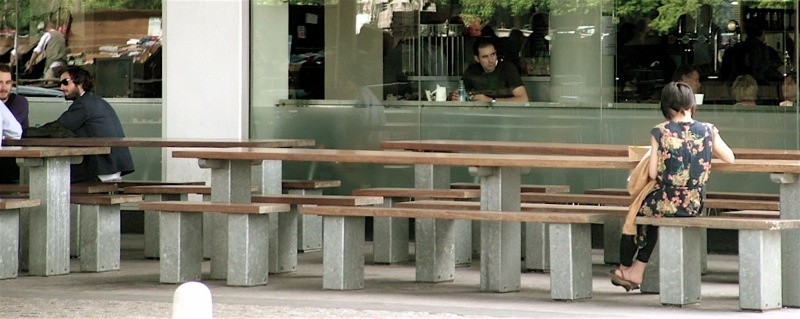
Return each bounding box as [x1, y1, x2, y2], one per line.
[1, 178, 800, 312]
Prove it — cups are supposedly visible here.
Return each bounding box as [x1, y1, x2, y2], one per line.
[694, 94, 704, 104]
[431, 84, 447, 101]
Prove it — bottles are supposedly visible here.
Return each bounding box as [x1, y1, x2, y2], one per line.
[766, 12, 778, 30]
[458, 80, 466, 101]
[527, 51, 550, 75]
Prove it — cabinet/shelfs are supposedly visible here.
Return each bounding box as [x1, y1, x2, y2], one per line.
[95, 56, 162, 99]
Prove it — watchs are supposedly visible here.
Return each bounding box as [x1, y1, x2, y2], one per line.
[492, 97, 496, 102]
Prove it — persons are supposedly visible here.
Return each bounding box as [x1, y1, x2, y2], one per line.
[26, 22, 67, 84]
[608, 80, 734, 292]
[450, 37, 529, 103]
[672, 2, 797, 109]
[473, 12, 549, 58]
[0, 63, 29, 181]
[57, 65, 135, 182]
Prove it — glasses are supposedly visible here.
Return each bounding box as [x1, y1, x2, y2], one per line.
[58, 78, 78, 87]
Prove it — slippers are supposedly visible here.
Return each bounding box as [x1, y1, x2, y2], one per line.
[609, 268, 638, 289]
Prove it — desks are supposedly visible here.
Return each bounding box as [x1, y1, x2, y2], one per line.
[172, 148, 799, 306]
[0, 137, 316, 273]
[383, 138, 800, 310]
[1, 145, 109, 278]
